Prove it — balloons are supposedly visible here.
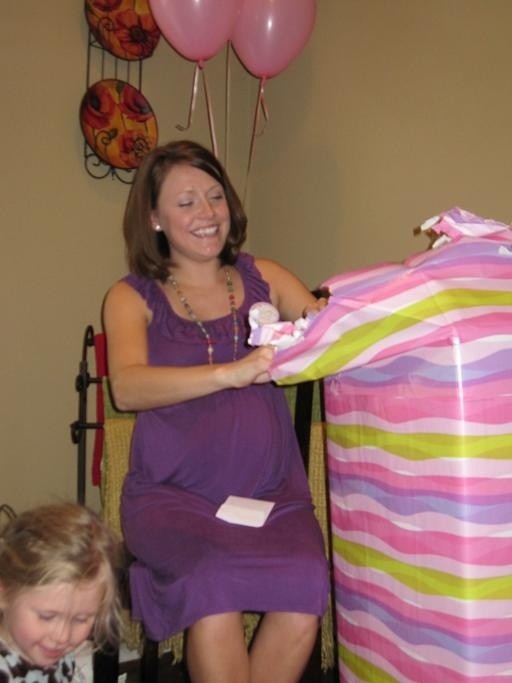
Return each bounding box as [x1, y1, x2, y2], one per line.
[149, 0, 229, 65]
[229, 0, 316, 82]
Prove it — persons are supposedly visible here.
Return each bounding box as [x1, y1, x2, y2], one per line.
[0, 501, 130, 682]
[101, 138, 329, 682]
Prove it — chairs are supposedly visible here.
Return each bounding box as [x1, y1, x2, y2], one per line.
[70, 326, 261, 683]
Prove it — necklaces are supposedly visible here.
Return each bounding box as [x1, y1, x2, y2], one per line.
[166, 268, 239, 363]
